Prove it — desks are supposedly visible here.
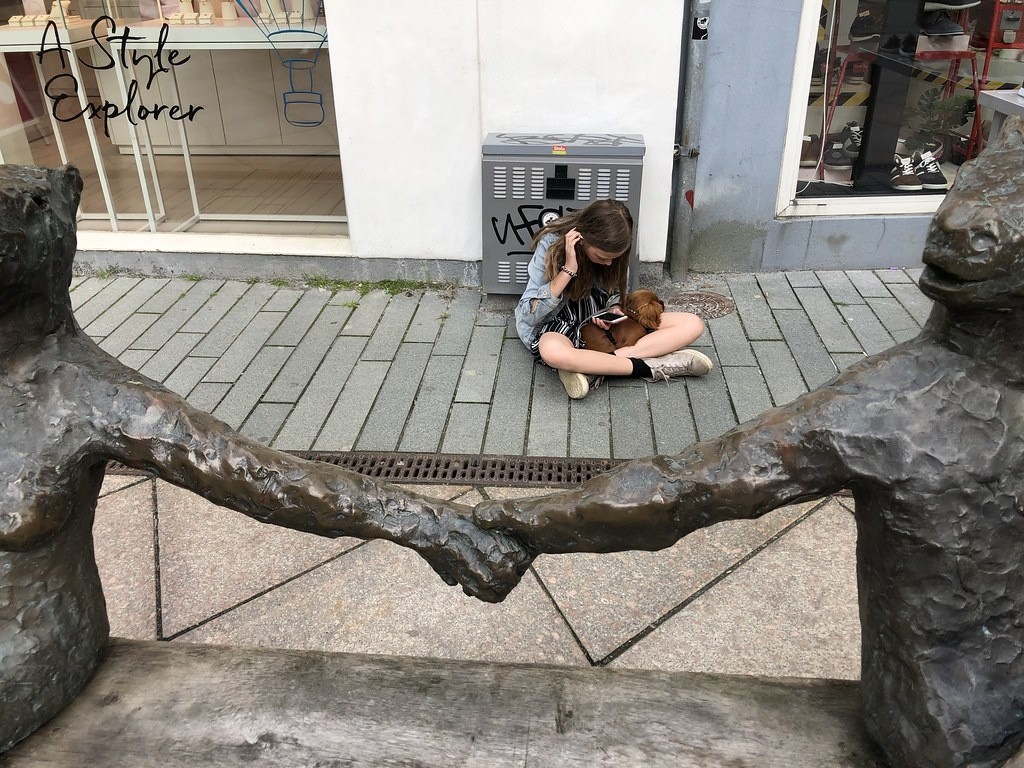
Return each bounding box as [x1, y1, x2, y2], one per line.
[106, 17, 347, 232]
[977, 90, 1024, 147]
[0, 18, 169, 232]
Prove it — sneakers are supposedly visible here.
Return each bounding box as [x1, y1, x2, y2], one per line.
[639, 349, 713, 387]
[820, 133, 852, 171]
[839, 120, 863, 158]
[557, 369, 605, 400]
[910, 150, 949, 189]
[888, 154, 922, 191]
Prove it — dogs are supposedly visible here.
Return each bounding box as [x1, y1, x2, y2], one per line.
[579, 288, 664, 354]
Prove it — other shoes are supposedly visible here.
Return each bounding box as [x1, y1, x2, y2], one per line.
[969, 34, 989, 51]
[879, 35, 901, 53]
[922, 0, 982, 11]
[840, 59, 868, 77]
[812, 47, 841, 78]
[918, 9, 965, 36]
[848, 16, 884, 41]
[1017, 81, 1024, 97]
[899, 34, 916, 57]
[800, 133, 822, 167]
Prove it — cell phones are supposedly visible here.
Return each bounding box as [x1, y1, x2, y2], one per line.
[592, 312, 628, 324]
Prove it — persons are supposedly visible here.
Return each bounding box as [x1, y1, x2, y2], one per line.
[514, 197, 713, 399]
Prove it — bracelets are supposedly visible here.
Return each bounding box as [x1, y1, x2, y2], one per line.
[560, 265, 576, 277]
